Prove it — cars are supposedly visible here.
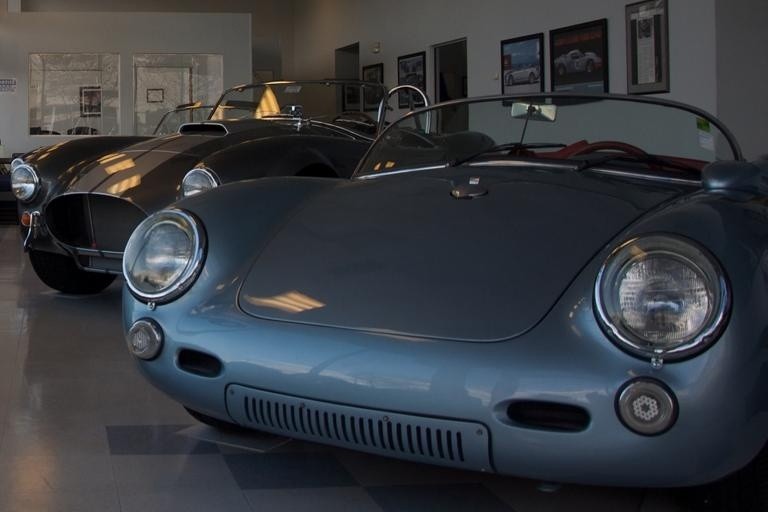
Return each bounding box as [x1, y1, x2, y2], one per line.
[504, 63, 540, 86]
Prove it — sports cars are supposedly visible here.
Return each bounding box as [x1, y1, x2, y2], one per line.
[120, 89, 768, 512]
[9, 78, 437, 294]
[554, 50, 603, 77]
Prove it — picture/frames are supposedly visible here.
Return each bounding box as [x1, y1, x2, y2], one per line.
[340, 51, 427, 114]
[500, 0, 670, 107]
[146, 88, 164, 104]
[79, 86, 102, 118]
